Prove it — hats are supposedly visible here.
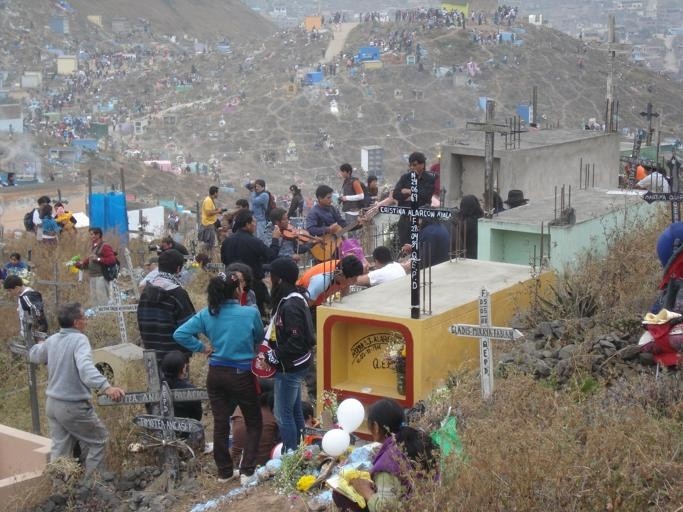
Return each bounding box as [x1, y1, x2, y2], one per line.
[159, 350, 187, 376]
[503, 189, 528, 204]
[158, 249, 187, 267]
[261, 257, 299, 282]
[232, 210, 255, 234]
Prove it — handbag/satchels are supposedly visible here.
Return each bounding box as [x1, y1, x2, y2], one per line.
[249, 343, 279, 379]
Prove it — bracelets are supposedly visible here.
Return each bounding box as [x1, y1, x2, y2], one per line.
[97, 257, 99, 264]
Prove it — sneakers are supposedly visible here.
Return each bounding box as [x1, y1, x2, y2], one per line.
[216, 470, 240, 484]
[200, 441, 214, 456]
[240, 470, 262, 486]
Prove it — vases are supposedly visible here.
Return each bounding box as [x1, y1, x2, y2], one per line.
[395, 369, 405, 396]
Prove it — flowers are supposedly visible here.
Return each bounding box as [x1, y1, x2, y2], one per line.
[64, 254, 90, 283]
[381, 329, 406, 373]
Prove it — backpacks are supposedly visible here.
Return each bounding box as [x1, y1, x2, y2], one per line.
[264, 193, 277, 220]
[100, 251, 121, 281]
[355, 181, 372, 209]
[23, 213, 33, 231]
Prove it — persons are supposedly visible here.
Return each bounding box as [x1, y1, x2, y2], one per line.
[0, 253, 31, 294]
[27, 303, 122, 486]
[634, 161, 673, 200]
[3, 171, 15, 187]
[73, 226, 120, 306]
[2, 275, 47, 365]
[317, 5, 518, 77]
[141, 151, 528, 485]
[8, 27, 203, 142]
[24, 196, 75, 254]
[333, 399, 444, 510]
